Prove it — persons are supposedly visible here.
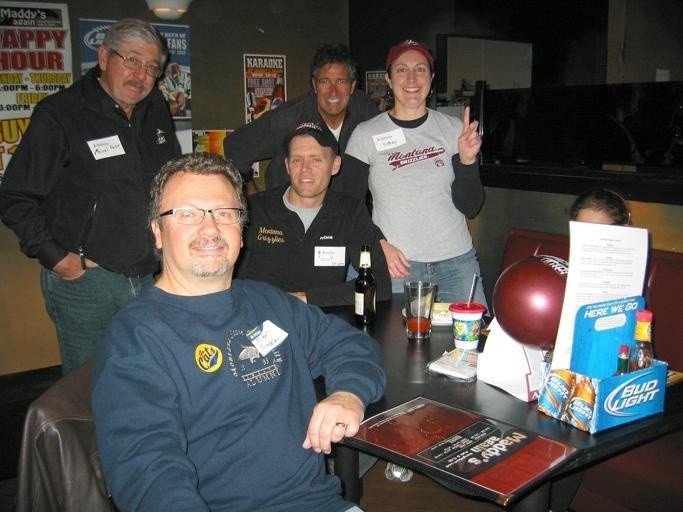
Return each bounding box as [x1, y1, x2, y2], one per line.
[1, 17, 181, 379]
[223, 42, 382, 217]
[485, 87, 536, 162]
[233, 122, 392, 306]
[569, 187, 630, 226]
[340, 38, 492, 318]
[90, 151, 387, 512]
[546, 84, 681, 168]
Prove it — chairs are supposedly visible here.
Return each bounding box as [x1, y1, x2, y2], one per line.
[15, 356, 118, 512]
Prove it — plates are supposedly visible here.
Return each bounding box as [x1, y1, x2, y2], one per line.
[401, 302, 453, 326]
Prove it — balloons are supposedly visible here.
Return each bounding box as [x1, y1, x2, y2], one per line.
[492, 253, 570, 346]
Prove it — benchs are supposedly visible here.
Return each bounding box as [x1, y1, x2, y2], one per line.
[501, 229, 683, 511]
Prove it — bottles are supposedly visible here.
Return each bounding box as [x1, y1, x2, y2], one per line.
[629, 310, 654, 371]
[563, 376, 595, 431]
[538, 368, 575, 421]
[354, 244, 378, 325]
[612, 345, 630, 377]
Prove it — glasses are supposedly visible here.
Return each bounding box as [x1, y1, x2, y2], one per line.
[108, 47, 163, 79]
[153, 207, 243, 226]
[310, 75, 352, 86]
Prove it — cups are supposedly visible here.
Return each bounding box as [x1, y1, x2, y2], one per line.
[448, 302, 486, 350]
[404, 281, 437, 339]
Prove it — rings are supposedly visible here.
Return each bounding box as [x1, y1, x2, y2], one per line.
[335, 422, 345, 428]
[474, 130, 480, 137]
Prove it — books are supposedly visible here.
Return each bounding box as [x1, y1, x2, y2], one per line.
[337, 395, 580, 507]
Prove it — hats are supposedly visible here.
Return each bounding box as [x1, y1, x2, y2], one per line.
[283, 123, 339, 156]
[385, 38, 434, 74]
